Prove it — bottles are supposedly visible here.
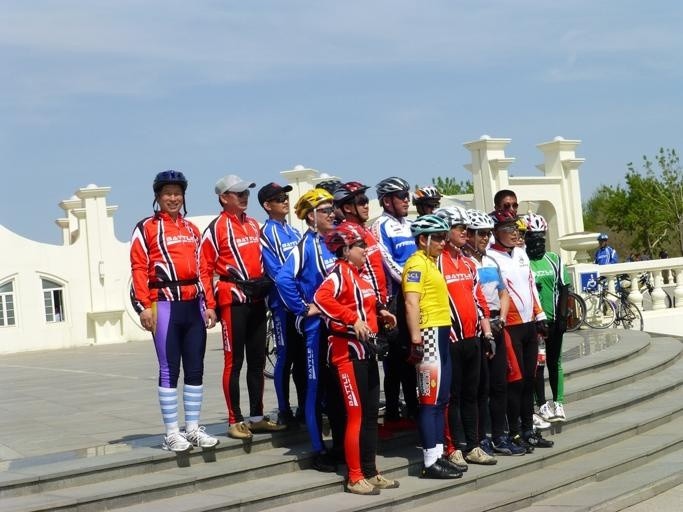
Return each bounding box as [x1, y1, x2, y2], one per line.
[538, 332, 546, 368]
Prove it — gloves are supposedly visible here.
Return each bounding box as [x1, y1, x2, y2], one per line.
[484, 336, 496, 353]
[535, 320, 549, 338]
[407, 343, 424, 365]
[490, 319, 505, 338]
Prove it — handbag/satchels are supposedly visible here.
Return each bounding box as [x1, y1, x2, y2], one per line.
[243, 274, 275, 299]
[620, 279, 631, 294]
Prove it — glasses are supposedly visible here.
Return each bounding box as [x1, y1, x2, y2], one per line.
[227, 190, 250, 197]
[267, 195, 288, 203]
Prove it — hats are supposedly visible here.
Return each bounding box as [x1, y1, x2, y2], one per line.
[215, 175, 256, 194]
[259, 183, 292, 201]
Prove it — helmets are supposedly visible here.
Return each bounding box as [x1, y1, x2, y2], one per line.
[153, 170, 187, 194]
[598, 234, 608, 240]
[295, 177, 548, 253]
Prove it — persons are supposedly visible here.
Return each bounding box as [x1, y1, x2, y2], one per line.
[593, 234, 618, 292]
[198, 175, 570, 495]
[658, 247, 669, 259]
[625, 251, 650, 261]
[129, 170, 221, 453]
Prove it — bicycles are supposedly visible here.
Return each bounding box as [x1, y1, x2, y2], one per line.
[621, 273, 671, 310]
[261, 311, 296, 381]
[560, 284, 585, 332]
[573, 277, 642, 332]
